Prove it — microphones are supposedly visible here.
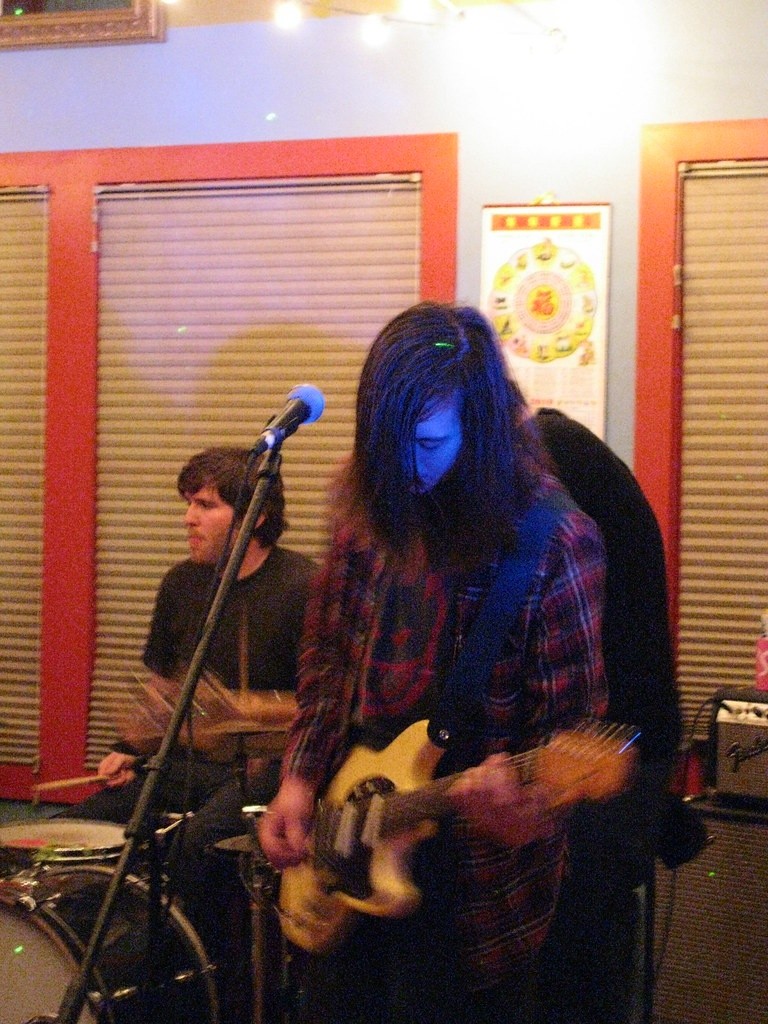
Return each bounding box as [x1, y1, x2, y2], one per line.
[250, 384, 324, 458]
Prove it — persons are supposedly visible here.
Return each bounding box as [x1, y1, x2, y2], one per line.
[257, 300, 685, 1024]
[45, 446, 324, 1024]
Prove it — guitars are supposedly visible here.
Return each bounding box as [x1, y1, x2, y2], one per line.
[272, 714, 643, 956]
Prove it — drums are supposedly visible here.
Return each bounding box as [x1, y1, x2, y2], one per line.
[0, 865, 222, 1024]
[1, 817, 133, 875]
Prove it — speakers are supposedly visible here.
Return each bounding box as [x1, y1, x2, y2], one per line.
[641, 791, 768, 1024]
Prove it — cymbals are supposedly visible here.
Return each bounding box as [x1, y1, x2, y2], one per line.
[214, 833, 260, 853]
[109, 719, 294, 764]
